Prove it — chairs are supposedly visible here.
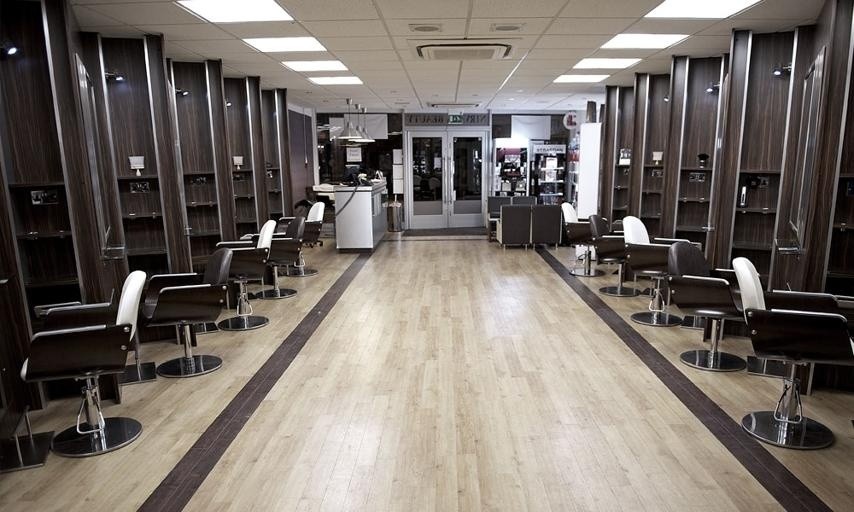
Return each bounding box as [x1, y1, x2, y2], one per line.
[277, 200, 326, 278]
[238, 216, 310, 300]
[139, 246, 233, 380]
[511, 195, 539, 205]
[667, 238, 747, 373]
[560, 201, 608, 279]
[732, 258, 854, 449]
[531, 205, 560, 251]
[622, 216, 691, 329]
[487, 196, 512, 243]
[588, 214, 640, 297]
[494, 204, 530, 250]
[214, 218, 278, 332]
[21, 269, 146, 461]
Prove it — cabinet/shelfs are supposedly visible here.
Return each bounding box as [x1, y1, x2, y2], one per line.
[531, 140, 566, 202]
[568, 123, 600, 218]
[492, 139, 527, 194]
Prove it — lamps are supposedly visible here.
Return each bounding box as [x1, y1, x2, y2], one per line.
[357, 118, 370, 141]
[181, 87, 190, 96]
[115, 74, 125, 83]
[337, 122, 362, 142]
[662, 92, 670, 103]
[774, 62, 791, 77]
[226, 99, 235, 109]
[3, 40, 19, 60]
[365, 108, 377, 142]
[705, 79, 721, 94]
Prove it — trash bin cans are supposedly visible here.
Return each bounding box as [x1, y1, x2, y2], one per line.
[387, 202, 402, 232]
